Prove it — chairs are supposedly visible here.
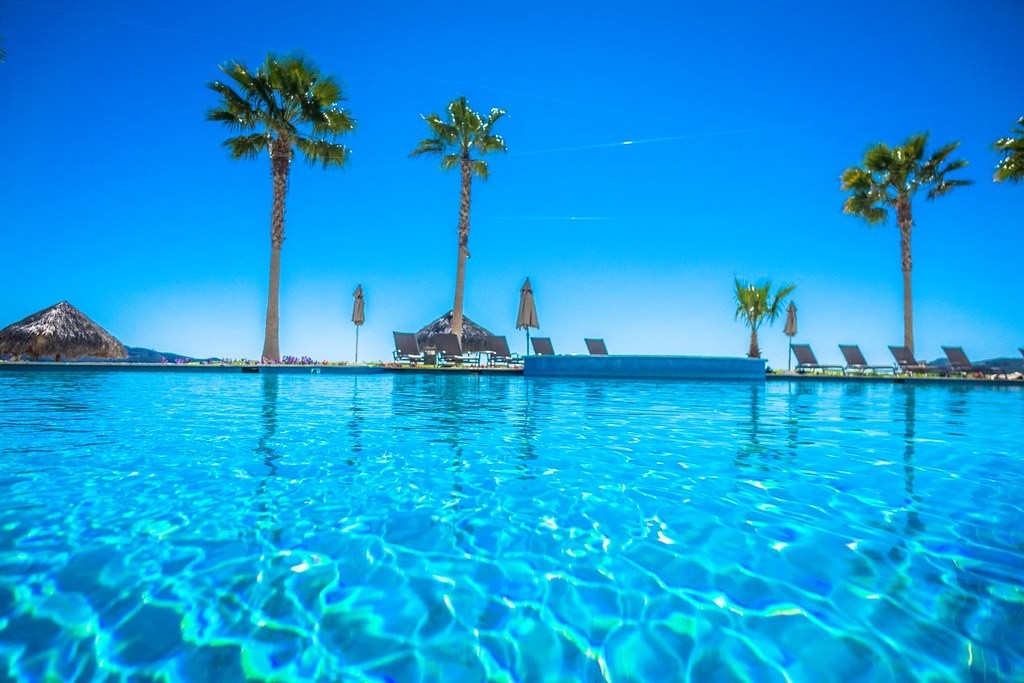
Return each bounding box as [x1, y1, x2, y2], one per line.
[392, 331, 433, 367]
[434, 333, 480, 367]
[942, 346, 1009, 379]
[479, 335, 524, 367]
[887, 345, 950, 377]
[584, 338, 608, 354]
[791, 345, 846, 375]
[530, 337, 555, 354]
[838, 344, 896, 376]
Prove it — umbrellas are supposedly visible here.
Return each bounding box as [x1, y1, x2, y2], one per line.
[397, 311, 497, 356]
[783, 300, 798, 371]
[352, 284, 365, 363]
[516, 278, 540, 356]
[0, 300, 129, 360]
